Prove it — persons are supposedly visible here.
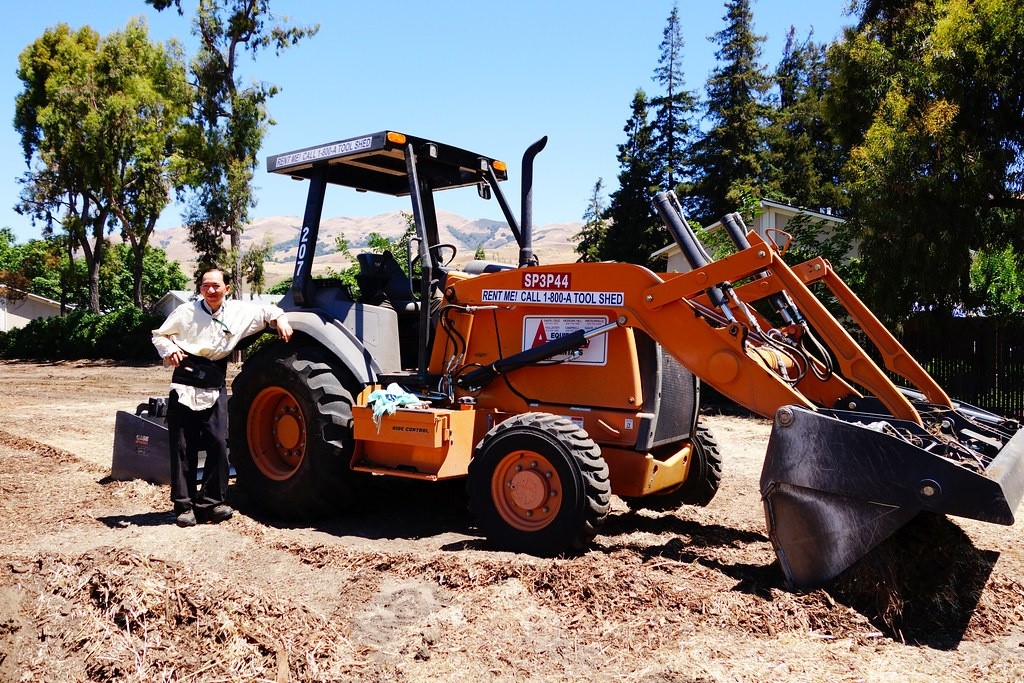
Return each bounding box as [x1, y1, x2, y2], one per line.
[151, 269, 294, 526]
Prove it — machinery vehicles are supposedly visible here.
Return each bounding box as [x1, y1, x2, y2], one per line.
[110, 130, 1024, 593]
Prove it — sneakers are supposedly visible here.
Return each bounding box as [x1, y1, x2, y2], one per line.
[177, 509, 196, 527]
[208, 504, 233, 520]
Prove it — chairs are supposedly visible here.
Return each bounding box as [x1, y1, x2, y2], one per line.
[356, 251, 423, 315]
